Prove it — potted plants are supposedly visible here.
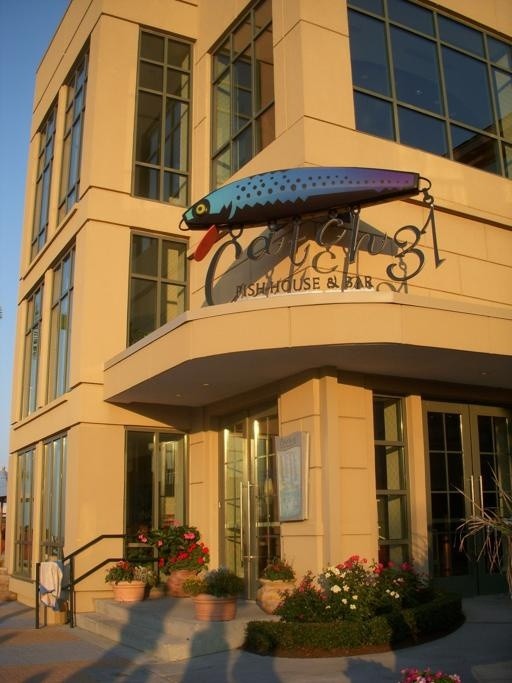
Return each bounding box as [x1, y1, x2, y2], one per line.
[183, 564, 245, 621]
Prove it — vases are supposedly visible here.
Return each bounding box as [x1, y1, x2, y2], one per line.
[110, 581, 147, 601]
[255, 577, 297, 615]
[166, 570, 198, 597]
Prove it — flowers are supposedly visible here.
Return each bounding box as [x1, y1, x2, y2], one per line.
[136, 524, 209, 575]
[105, 561, 159, 587]
[261, 557, 295, 582]
